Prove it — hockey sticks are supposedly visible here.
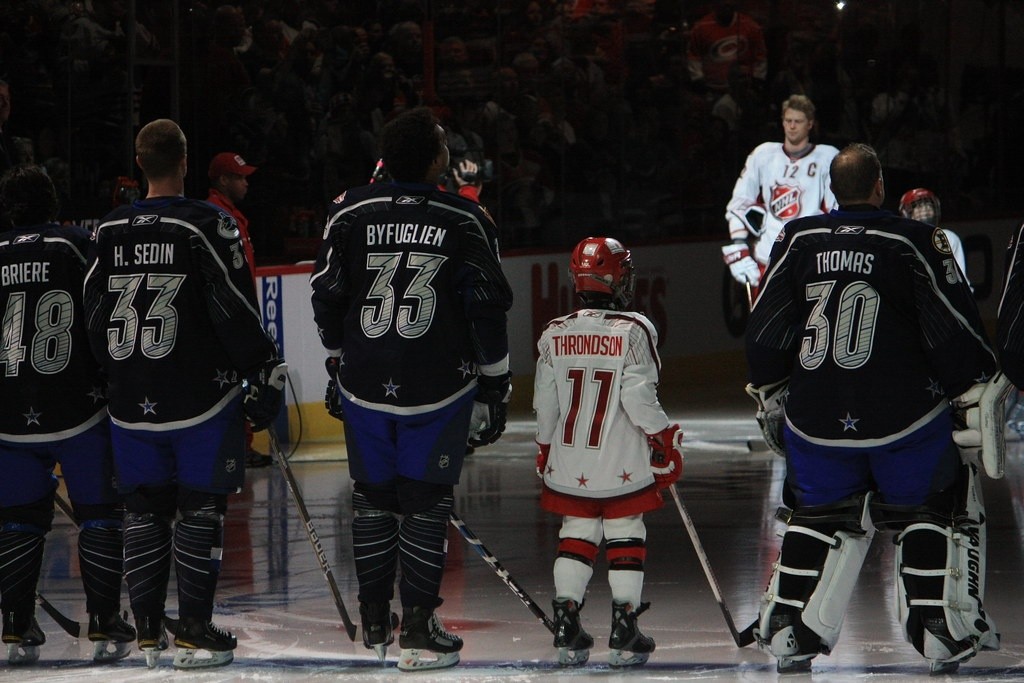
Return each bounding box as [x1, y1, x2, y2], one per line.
[449, 511, 554, 631]
[50, 493, 79, 524]
[669, 483, 760, 647]
[33, 591, 126, 638]
[267, 422, 399, 642]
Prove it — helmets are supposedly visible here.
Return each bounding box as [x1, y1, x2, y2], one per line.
[898, 188, 942, 227]
[570, 236, 636, 311]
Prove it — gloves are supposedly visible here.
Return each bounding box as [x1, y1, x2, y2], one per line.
[648, 423, 684, 489]
[241, 359, 288, 432]
[534, 438, 551, 478]
[324, 356, 343, 421]
[453, 160, 478, 186]
[465, 370, 512, 455]
[745, 377, 791, 458]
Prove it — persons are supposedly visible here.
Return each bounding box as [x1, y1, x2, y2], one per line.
[723, 94, 841, 309]
[531, 238, 687, 666]
[207, 151, 278, 470]
[0, 168, 137, 659]
[757, 144, 1023, 683]
[893, 182, 968, 283]
[83, 120, 278, 668]
[443, 151, 493, 192]
[309, 116, 513, 676]
[0, 0, 1023, 247]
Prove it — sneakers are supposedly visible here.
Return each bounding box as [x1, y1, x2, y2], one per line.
[551, 598, 594, 667]
[2, 603, 45, 664]
[173, 615, 237, 670]
[721, 243, 761, 288]
[244, 447, 273, 469]
[359, 602, 395, 669]
[607, 600, 655, 669]
[771, 614, 818, 674]
[397, 605, 463, 672]
[919, 616, 977, 676]
[88, 606, 137, 661]
[136, 614, 169, 669]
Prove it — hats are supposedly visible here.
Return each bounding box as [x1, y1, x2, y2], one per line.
[208, 152, 257, 180]
